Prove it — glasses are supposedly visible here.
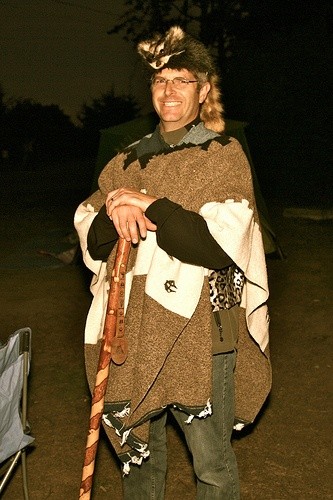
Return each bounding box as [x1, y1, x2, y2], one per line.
[151, 76, 201, 88]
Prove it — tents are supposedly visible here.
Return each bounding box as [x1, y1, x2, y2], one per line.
[42, 111, 288, 271]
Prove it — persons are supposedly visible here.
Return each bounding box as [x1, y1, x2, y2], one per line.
[73, 25, 274, 500]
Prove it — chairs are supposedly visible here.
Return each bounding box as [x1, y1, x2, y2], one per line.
[0, 325, 36, 500]
